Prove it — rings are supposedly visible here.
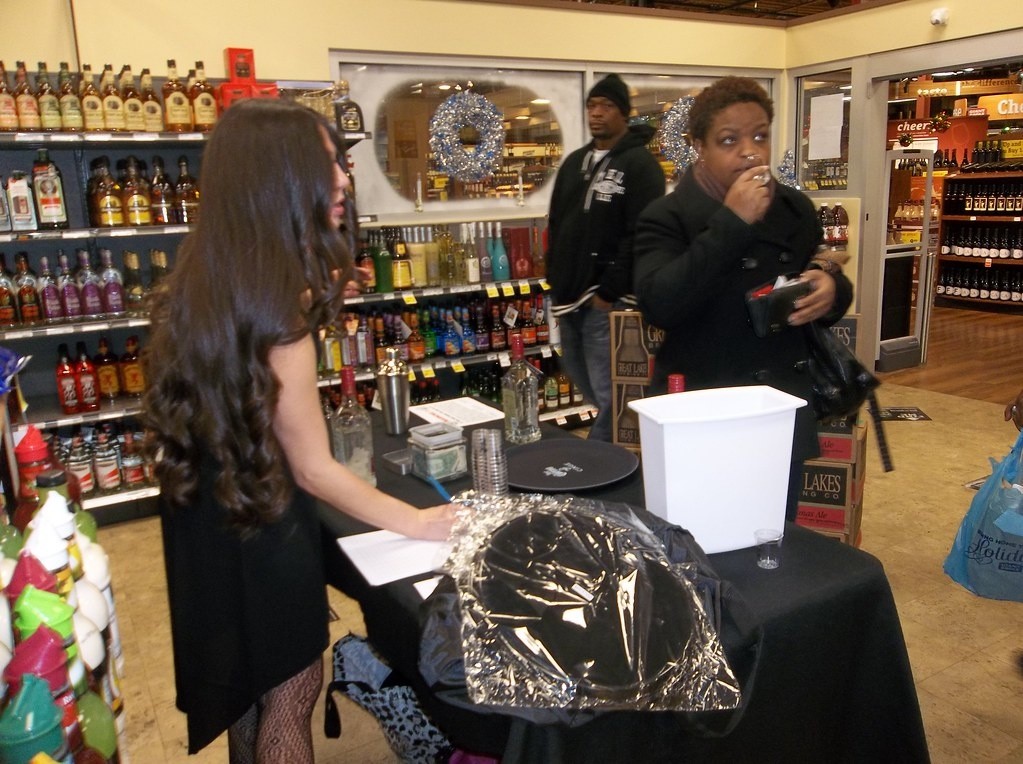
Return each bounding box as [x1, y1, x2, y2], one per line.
[1012, 405, 1017, 420]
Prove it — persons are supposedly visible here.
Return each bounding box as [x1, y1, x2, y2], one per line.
[633, 75, 854, 524]
[136, 96, 471, 764]
[1004, 388, 1023, 432]
[543, 72, 665, 443]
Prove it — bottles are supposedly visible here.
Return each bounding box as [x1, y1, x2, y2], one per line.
[0, 56, 618, 764]
[667, 373, 686, 395]
[886, 136, 1023, 306]
[816, 201, 850, 244]
[801, 153, 850, 193]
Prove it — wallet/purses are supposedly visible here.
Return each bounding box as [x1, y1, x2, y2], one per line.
[745, 271, 813, 339]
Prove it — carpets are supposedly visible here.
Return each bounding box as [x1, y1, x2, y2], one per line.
[90, 365, 1023, 764]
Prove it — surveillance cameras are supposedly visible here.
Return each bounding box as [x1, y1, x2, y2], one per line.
[929, 9, 948, 26]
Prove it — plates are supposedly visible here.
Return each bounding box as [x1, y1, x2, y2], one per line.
[503, 436, 639, 492]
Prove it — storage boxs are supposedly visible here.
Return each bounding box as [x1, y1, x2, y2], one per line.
[406, 422, 468, 482]
[0, 52, 1023, 512]
[606, 311, 870, 555]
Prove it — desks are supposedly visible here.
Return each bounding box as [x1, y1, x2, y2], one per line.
[310, 412, 717, 764]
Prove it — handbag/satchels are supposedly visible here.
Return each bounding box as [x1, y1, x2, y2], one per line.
[809, 318, 894, 422]
[943, 428, 1023, 603]
[325, 631, 457, 764]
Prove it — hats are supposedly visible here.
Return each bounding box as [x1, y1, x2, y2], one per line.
[585, 73, 631, 116]
